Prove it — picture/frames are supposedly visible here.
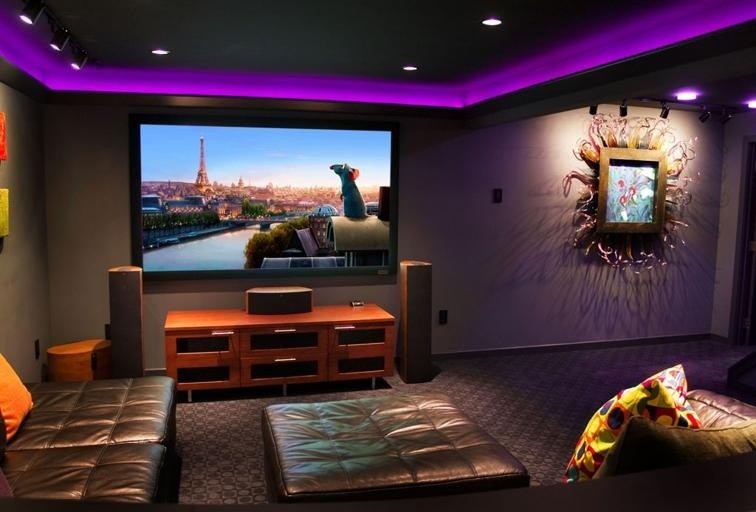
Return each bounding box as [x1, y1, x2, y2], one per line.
[596, 146, 668, 235]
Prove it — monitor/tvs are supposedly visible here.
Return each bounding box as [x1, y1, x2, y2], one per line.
[126, 112, 399, 280]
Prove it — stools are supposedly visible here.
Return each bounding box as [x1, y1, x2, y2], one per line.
[44, 339, 111, 386]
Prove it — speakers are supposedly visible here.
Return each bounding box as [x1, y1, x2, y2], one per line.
[398, 261, 434, 384]
[244, 286, 313, 315]
[109, 264, 144, 377]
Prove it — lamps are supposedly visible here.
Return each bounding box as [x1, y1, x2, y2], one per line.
[589, 97, 733, 125]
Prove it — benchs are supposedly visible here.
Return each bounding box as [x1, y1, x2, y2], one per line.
[259, 394, 531, 502]
[3, 372, 177, 506]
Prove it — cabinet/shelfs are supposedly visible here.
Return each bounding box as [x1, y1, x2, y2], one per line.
[160, 303, 398, 403]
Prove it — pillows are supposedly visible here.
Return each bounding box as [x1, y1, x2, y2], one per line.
[561, 361, 756, 484]
